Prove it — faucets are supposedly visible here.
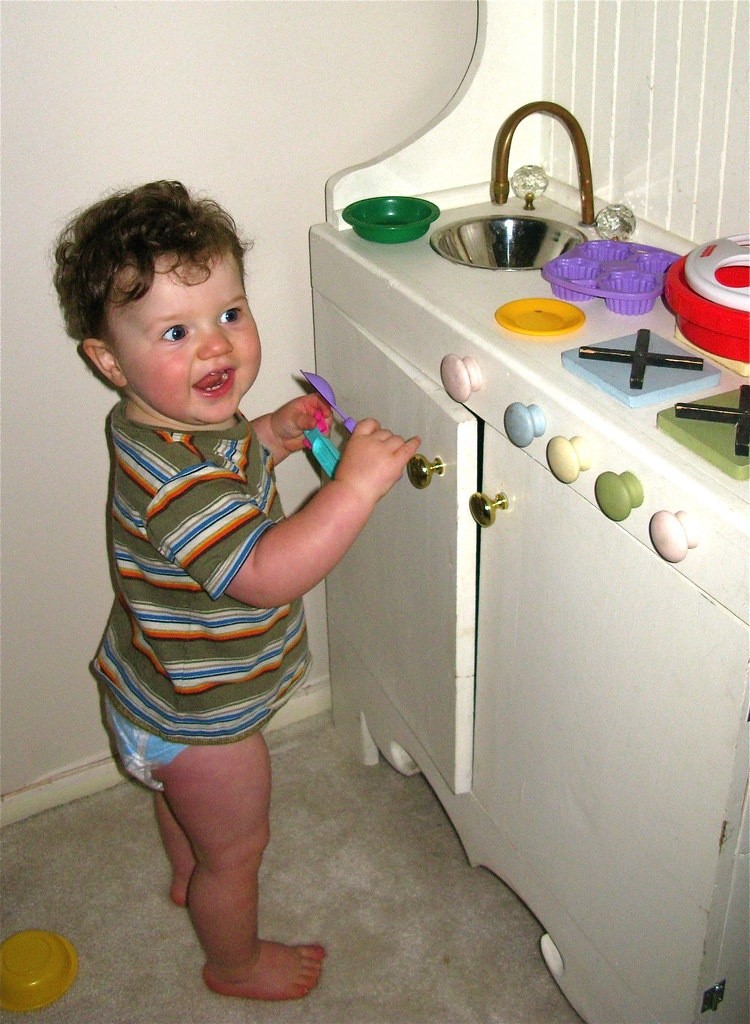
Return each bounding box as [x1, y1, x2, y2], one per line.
[491, 100, 596, 227]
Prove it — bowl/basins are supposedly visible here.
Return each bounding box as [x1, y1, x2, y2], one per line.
[342, 196, 441, 244]
[0, 929, 79, 1013]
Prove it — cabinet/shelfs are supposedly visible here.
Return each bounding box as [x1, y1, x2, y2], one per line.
[309, 226, 750, 1024]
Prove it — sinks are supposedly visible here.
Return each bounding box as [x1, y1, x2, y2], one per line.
[430, 214, 588, 271]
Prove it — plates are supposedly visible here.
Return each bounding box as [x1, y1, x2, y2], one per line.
[496, 298, 586, 337]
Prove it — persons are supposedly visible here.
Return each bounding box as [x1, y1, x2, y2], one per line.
[54, 180, 422, 1000]
